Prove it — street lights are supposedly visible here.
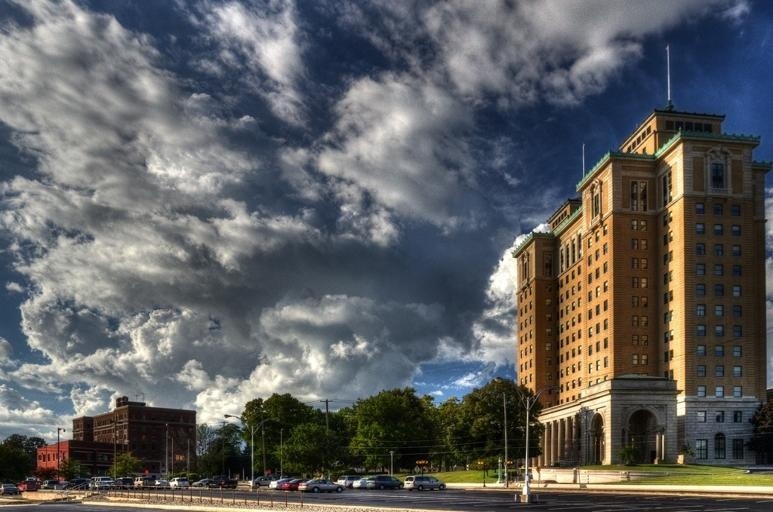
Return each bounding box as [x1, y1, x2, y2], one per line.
[222, 413, 280, 491]
[56, 426, 66, 481]
[515, 383, 562, 503]
[389, 450, 395, 477]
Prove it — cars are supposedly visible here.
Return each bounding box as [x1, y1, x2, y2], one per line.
[0, 475, 238, 495]
[252, 472, 452, 493]
